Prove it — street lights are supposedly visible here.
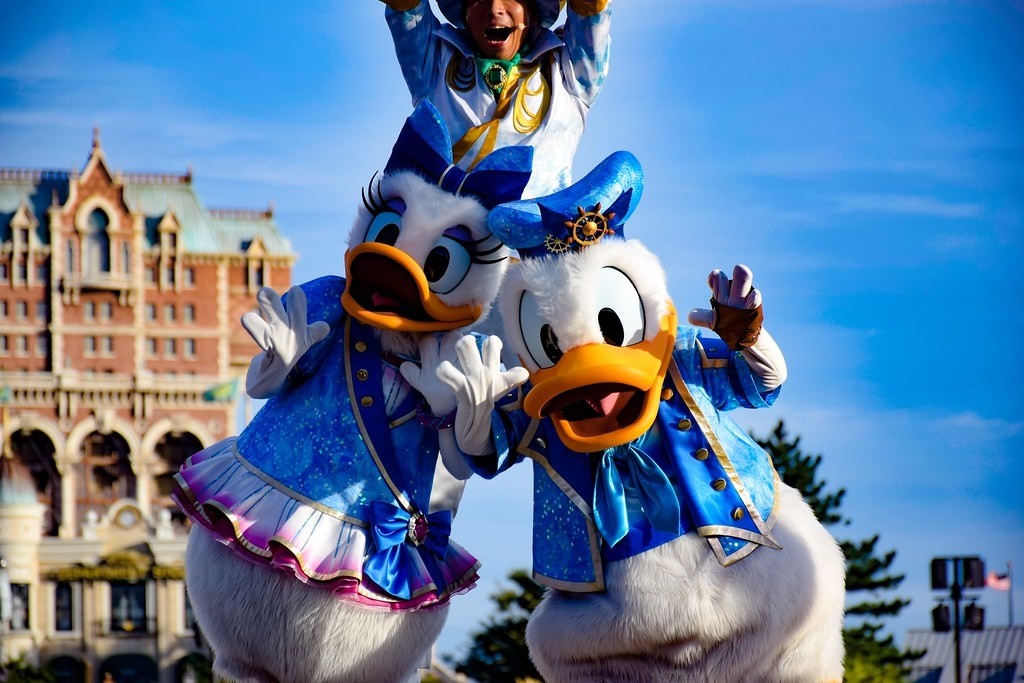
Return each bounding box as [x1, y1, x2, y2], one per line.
[930, 552, 987, 683]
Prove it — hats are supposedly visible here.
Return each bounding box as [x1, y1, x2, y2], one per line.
[434, 0, 567, 29]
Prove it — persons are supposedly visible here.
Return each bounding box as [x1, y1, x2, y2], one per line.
[376, 0, 612, 202]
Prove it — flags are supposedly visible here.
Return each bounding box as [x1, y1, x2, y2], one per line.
[986, 571, 1010, 591]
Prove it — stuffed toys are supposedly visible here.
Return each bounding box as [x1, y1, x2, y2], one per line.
[171, 99, 533, 683]
[436, 151, 847, 683]
